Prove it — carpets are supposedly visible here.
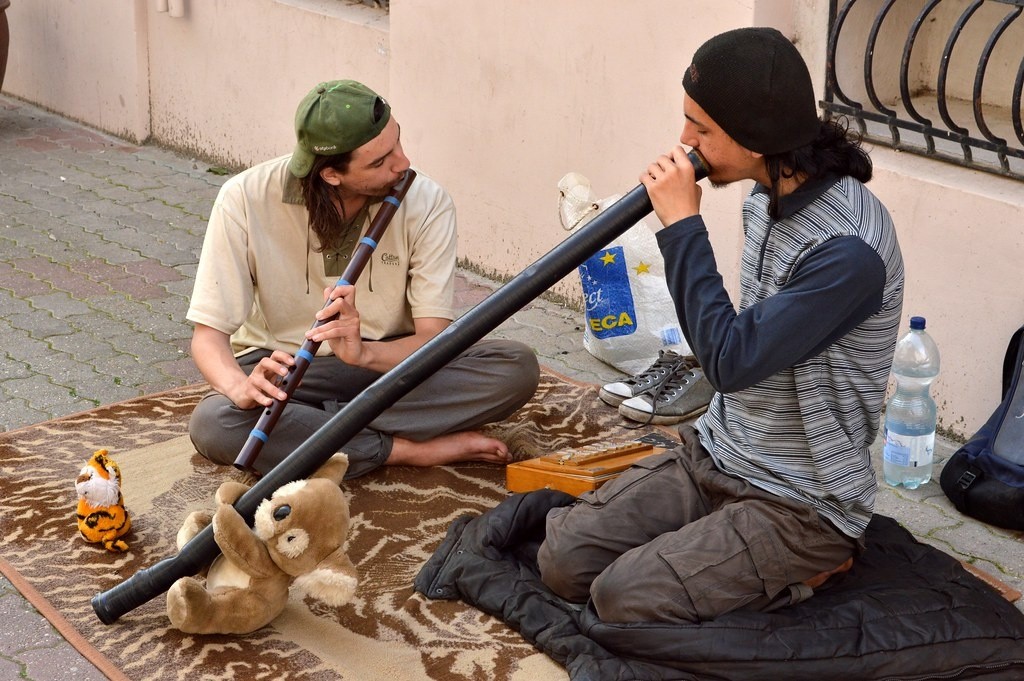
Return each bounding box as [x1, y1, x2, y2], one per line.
[0, 366, 1024, 681]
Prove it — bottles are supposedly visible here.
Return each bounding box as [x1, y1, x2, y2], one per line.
[882, 317, 941, 491]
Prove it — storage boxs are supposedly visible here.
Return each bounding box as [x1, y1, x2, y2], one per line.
[507, 441, 670, 497]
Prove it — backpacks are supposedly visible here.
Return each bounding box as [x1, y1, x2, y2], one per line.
[939, 325, 1024, 529]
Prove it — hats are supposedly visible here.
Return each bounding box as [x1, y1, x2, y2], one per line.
[681, 26, 822, 156]
[287, 79, 391, 179]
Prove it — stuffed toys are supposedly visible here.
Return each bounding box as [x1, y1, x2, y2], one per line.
[74, 449, 131, 552]
[167, 453, 359, 634]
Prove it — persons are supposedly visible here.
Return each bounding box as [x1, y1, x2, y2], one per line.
[537, 28, 904, 629]
[188, 79, 539, 478]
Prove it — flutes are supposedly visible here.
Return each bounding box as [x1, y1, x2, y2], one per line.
[225, 161, 424, 480]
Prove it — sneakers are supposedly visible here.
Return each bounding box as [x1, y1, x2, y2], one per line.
[619, 355, 716, 427]
[598, 350, 684, 408]
